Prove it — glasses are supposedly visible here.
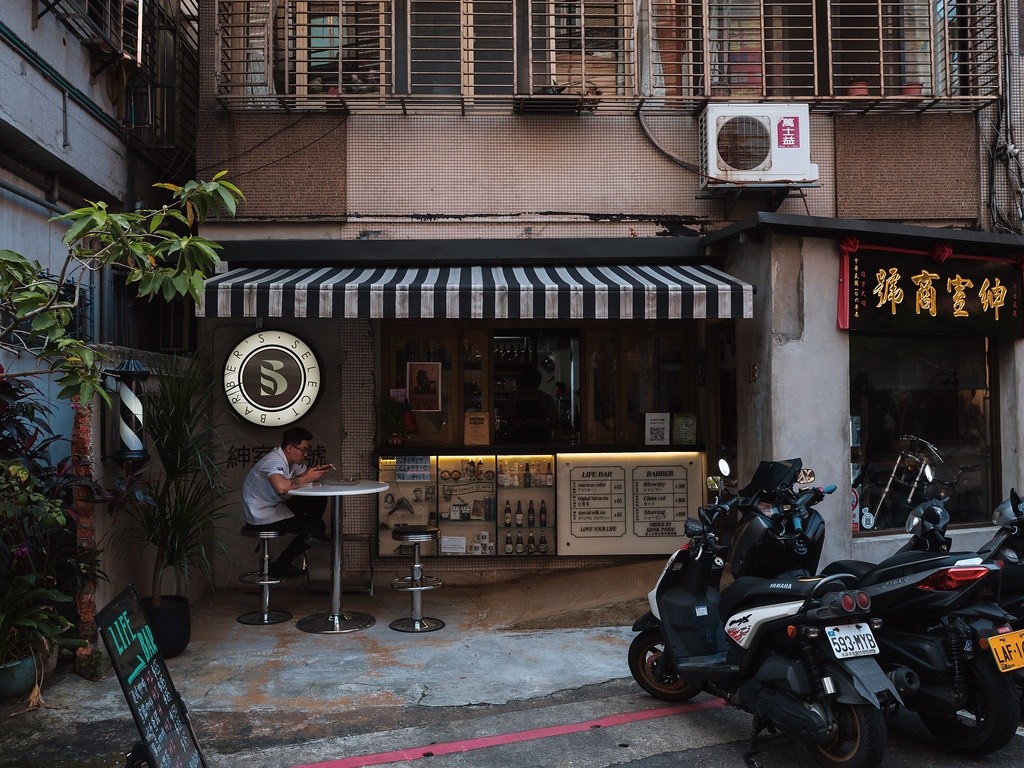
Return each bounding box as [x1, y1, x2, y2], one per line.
[289, 443, 310, 455]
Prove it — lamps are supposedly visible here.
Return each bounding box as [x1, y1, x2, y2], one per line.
[101, 349, 150, 476]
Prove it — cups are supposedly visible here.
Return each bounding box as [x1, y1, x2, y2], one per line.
[467, 542, 482, 555]
[481, 543, 495, 554]
[475, 531, 490, 544]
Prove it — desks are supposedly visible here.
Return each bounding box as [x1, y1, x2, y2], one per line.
[287, 479, 390, 635]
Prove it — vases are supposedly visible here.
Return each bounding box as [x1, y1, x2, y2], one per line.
[900, 82, 923, 94]
[845, 82, 869, 96]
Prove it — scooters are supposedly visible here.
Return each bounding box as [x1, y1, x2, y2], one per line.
[852, 435, 989, 526]
[629, 459, 922, 768]
[886, 486, 1024, 621]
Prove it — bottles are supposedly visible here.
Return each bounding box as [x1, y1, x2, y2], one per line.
[494, 343, 522, 370]
[504, 500, 511, 527]
[495, 381, 515, 407]
[528, 530, 535, 553]
[498, 460, 553, 488]
[539, 529, 547, 554]
[516, 530, 523, 554]
[466, 460, 483, 481]
[515, 500, 523, 527]
[528, 499, 535, 527]
[505, 530, 513, 554]
[540, 499, 546, 527]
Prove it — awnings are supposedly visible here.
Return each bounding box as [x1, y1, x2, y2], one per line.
[191, 264, 758, 318]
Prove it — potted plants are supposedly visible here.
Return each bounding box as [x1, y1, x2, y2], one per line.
[0, 362, 110, 699]
[90, 348, 247, 660]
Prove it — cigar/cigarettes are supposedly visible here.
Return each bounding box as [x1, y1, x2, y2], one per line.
[330, 464, 336, 471]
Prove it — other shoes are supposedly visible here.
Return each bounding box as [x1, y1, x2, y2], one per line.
[305, 532, 331, 548]
[267, 562, 306, 579]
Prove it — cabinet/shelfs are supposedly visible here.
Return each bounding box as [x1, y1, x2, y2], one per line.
[490, 332, 538, 442]
[437, 476, 557, 557]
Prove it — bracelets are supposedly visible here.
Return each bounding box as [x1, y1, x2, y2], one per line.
[289, 478, 299, 489]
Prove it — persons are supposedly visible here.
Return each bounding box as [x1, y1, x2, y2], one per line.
[502, 368, 560, 445]
[552, 382, 568, 409]
[241, 427, 336, 578]
[864, 383, 929, 457]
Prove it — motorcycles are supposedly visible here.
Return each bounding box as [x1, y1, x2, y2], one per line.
[705, 455, 1024, 756]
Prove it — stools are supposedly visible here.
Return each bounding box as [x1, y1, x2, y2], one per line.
[237, 523, 293, 625]
[389, 525, 446, 633]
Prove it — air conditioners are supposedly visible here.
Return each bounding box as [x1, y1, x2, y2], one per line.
[699, 103, 819, 190]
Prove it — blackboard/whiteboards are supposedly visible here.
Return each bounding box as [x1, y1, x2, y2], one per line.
[96, 582, 210, 768]
